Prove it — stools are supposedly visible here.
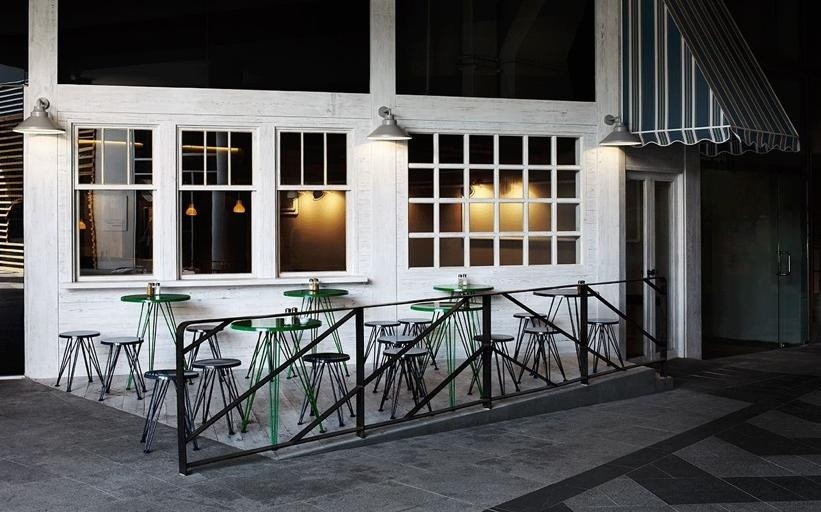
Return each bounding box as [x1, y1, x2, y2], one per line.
[138, 367, 200, 452]
[513, 312, 547, 362]
[298, 351, 356, 432]
[517, 327, 569, 387]
[186, 323, 223, 375]
[584, 315, 626, 375]
[53, 329, 104, 392]
[190, 356, 247, 437]
[468, 330, 523, 396]
[364, 313, 439, 421]
[98, 335, 147, 404]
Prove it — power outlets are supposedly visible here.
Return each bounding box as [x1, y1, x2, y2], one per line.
[365, 105, 414, 143]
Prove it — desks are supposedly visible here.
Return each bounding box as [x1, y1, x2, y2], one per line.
[229, 313, 328, 452]
[118, 289, 192, 393]
[431, 281, 495, 361]
[281, 286, 356, 381]
[531, 287, 599, 365]
[413, 300, 483, 407]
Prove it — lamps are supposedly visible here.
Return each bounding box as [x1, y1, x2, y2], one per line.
[184, 173, 197, 216]
[598, 114, 643, 146]
[8, 97, 67, 137]
[232, 171, 246, 213]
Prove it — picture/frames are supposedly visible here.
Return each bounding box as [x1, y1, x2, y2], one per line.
[99, 193, 131, 233]
